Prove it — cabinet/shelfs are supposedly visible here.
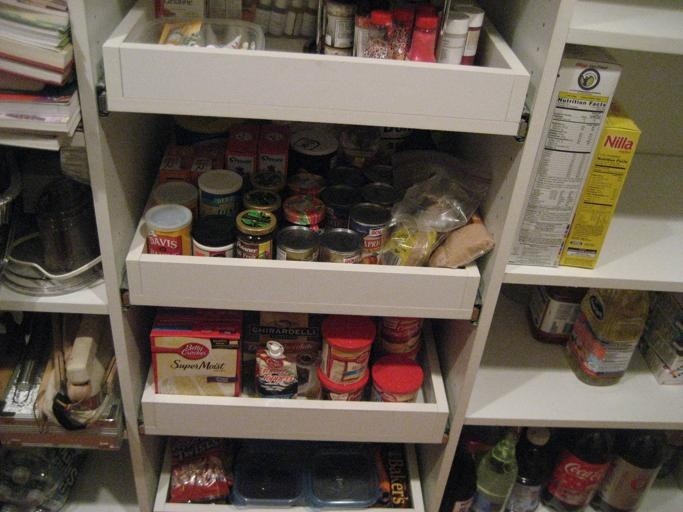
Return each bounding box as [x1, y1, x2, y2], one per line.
[0, 2, 143, 512]
[434, 0, 683, 512]
[63, 0, 580, 512]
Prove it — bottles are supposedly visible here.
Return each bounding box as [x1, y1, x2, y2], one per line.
[319, 1, 439, 62]
[289, 170, 323, 194]
[333, 165, 363, 185]
[544, 432, 613, 507]
[244, 189, 280, 209]
[462, 7, 485, 68]
[443, 14, 465, 66]
[509, 431, 551, 508]
[236, 207, 272, 261]
[284, 194, 325, 225]
[280, 223, 318, 260]
[477, 426, 517, 508]
[323, 227, 360, 262]
[325, 180, 355, 208]
[599, 425, 669, 507]
[247, 169, 280, 186]
[360, 180, 398, 203]
[352, 201, 392, 265]
[443, 432, 477, 508]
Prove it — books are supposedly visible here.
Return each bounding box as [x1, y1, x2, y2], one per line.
[0, 2, 83, 153]
